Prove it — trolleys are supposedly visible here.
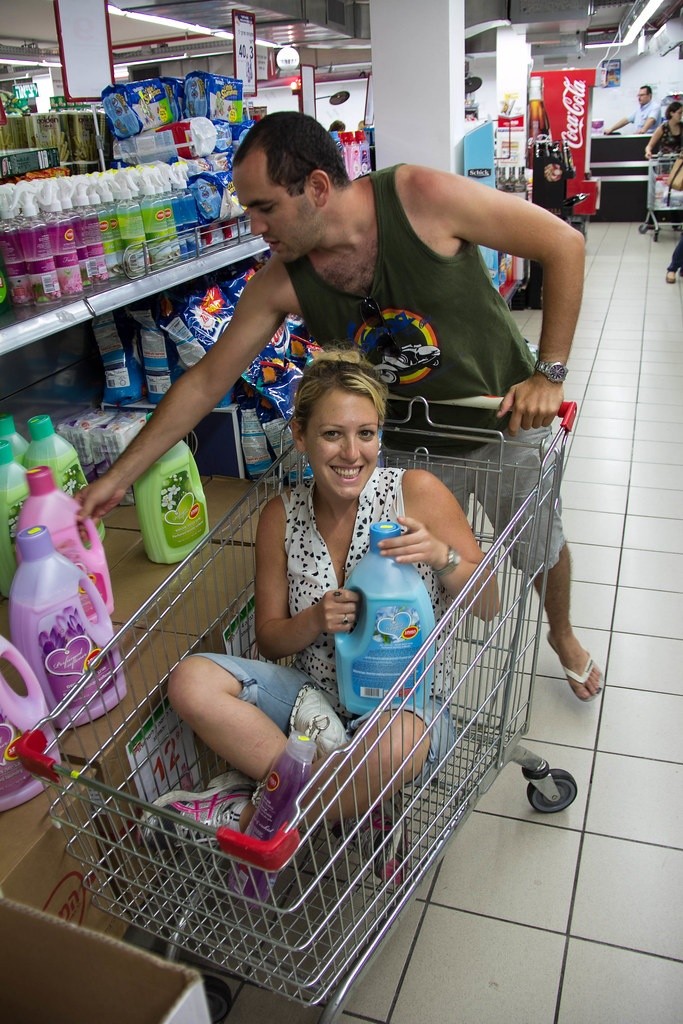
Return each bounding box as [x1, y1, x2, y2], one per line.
[12, 390, 583, 1024]
[639, 152, 683, 242]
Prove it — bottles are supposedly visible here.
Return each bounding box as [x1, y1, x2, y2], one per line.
[16, 465, 114, 624]
[0, 635, 62, 812]
[333, 522, 436, 716]
[9, 526, 127, 728]
[498, 167, 506, 192]
[0, 413, 30, 465]
[655, 177, 664, 207]
[133, 440, 209, 564]
[23, 414, 105, 549]
[515, 167, 525, 192]
[330, 130, 372, 182]
[526, 77, 553, 203]
[506, 167, 515, 192]
[0, 440, 31, 597]
[228, 731, 316, 909]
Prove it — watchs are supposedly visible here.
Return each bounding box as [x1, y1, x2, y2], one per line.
[431, 545, 461, 577]
[535, 360, 569, 383]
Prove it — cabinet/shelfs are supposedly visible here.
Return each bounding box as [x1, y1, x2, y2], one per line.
[0, 223, 292, 939]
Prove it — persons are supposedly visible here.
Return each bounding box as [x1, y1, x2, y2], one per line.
[666, 237, 683, 283]
[645, 101, 683, 228]
[137, 350, 501, 884]
[604, 86, 662, 135]
[74, 111, 604, 703]
[328, 120, 345, 132]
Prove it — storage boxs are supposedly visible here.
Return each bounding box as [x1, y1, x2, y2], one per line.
[0, 470, 272, 1024]
[0, 110, 110, 181]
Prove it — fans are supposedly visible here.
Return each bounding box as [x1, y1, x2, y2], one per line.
[465, 77, 483, 94]
[315, 92, 350, 104]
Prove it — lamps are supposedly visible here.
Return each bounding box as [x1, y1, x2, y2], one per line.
[584, 0, 663, 49]
[291, 82, 302, 95]
[0, 53, 61, 81]
[109, 5, 284, 65]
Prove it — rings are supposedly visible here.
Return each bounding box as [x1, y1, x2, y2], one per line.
[332, 591, 342, 597]
[342, 613, 348, 625]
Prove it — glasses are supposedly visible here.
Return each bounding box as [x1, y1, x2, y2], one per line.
[359, 297, 402, 357]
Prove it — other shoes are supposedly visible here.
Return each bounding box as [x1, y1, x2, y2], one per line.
[666, 272, 676, 283]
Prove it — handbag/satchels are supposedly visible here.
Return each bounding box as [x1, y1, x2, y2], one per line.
[666, 157, 683, 190]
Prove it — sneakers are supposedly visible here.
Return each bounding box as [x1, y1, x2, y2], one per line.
[331, 790, 412, 884]
[136, 770, 257, 851]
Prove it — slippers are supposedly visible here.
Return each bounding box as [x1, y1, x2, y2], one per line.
[545, 629, 603, 702]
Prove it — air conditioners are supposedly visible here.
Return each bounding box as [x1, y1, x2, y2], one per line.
[652, 17, 683, 57]
